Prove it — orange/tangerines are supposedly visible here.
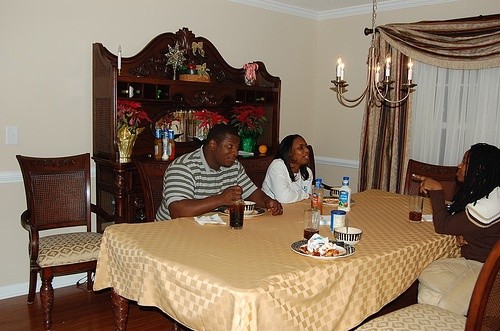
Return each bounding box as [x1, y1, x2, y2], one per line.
[259, 145, 267, 153]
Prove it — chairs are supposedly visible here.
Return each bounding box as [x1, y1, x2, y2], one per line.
[135, 161, 168, 222]
[17, 155, 103, 329]
[349, 240, 500, 331]
[405, 159, 459, 202]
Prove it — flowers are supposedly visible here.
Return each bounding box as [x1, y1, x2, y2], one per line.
[116, 100, 152, 129]
[230, 106, 267, 135]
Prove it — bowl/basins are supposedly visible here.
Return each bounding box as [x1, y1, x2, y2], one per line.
[331, 187, 341, 198]
[334, 227, 362, 247]
[226, 201, 256, 215]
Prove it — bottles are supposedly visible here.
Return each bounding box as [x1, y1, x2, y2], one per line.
[185, 65, 197, 75]
[167, 130, 175, 161]
[311, 179, 324, 215]
[154, 129, 163, 161]
[338, 177, 351, 212]
[162, 130, 168, 162]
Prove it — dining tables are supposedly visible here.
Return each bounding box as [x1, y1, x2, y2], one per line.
[94, 189, 463, 331]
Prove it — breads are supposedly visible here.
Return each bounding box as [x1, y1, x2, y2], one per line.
[325, 249, 339, 256]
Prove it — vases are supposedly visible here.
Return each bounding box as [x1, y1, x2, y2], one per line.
[116, 123, 144, 158]
[240, 136, 255, 152]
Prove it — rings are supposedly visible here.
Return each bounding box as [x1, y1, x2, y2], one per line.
[281, 209, 283, 212]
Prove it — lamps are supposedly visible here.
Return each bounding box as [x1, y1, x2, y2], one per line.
[331, 0, 417, 103]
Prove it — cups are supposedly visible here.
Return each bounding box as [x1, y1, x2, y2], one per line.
[409, 196, 424, 224]
[229, 199, 244, 229]
[304, 208, 320, 240]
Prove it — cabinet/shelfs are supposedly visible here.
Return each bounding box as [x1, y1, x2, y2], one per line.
[93, 27, 280, 232]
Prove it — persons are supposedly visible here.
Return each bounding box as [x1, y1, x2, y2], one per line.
[412, 142, 500, 315]
[260, 134, 313, 204]
[153, 124, 283, 223]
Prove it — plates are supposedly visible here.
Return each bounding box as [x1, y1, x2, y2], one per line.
[290, 240, 356, 259]
[215, 207, 265, 218]
[323, 197, 355, 205]
